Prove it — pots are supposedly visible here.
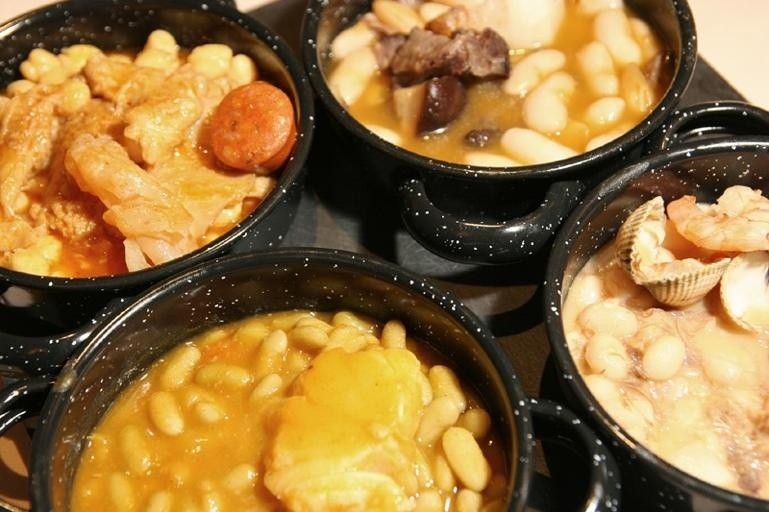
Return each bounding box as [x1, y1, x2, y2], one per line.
[546, 99, 769, 510]
[0, 241, 623, 511]
[0, 0, 322, 365]
[308, 0, 703, 266]
